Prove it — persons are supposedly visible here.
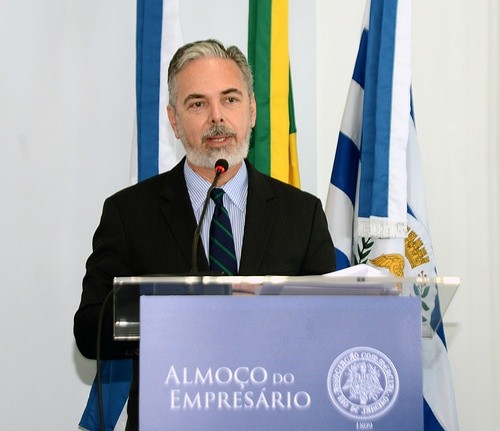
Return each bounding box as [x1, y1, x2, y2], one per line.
[72, 41, 337, 431]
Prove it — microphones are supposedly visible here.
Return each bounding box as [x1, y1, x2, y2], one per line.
[192, 159, 229, 276]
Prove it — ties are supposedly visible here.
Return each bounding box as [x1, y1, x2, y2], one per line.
[209, 188, 238, 276]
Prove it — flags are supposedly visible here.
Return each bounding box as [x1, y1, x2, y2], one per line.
[244, 0, 301, 192]
[323, 0, 459, 431]
[77, 0, 186, 431]
